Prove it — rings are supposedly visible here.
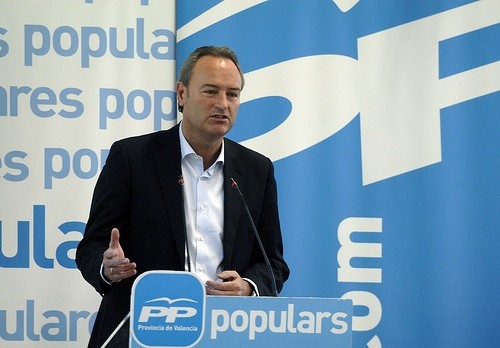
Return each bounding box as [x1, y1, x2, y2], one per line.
[111, 268, 113, 274]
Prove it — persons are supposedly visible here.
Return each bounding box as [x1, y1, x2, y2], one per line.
[75, 46, 289, 348]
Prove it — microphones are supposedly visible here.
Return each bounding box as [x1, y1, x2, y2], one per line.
[228, 176, 279, 297]
